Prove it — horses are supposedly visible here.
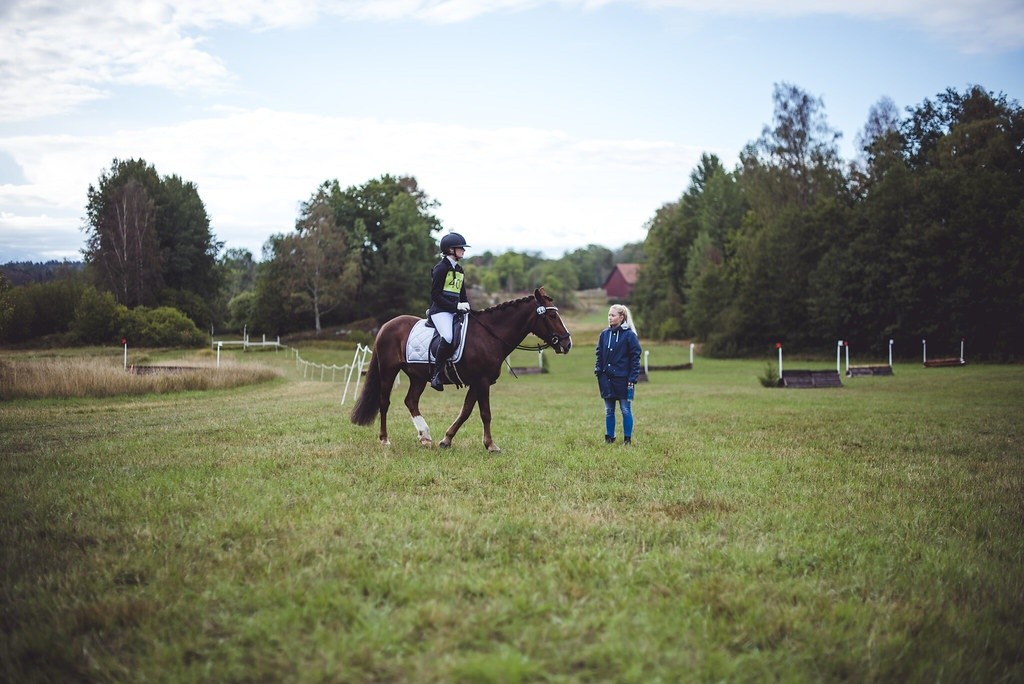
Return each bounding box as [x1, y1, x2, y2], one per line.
[350, 287, 572, 453]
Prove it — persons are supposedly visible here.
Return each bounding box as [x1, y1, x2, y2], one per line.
[594, 304, 642, 446]
[429, 232, 471, 391]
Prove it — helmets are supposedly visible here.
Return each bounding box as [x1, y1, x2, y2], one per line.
[441, 233, 471, 253]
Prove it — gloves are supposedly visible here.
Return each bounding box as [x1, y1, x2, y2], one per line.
[457, 302, 470, 312]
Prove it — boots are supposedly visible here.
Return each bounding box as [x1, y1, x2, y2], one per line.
[431, 337, 452, 391]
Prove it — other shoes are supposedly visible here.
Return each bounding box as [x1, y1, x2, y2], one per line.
[622, 436, 631, 445]
[605, 435, 615, 443]
